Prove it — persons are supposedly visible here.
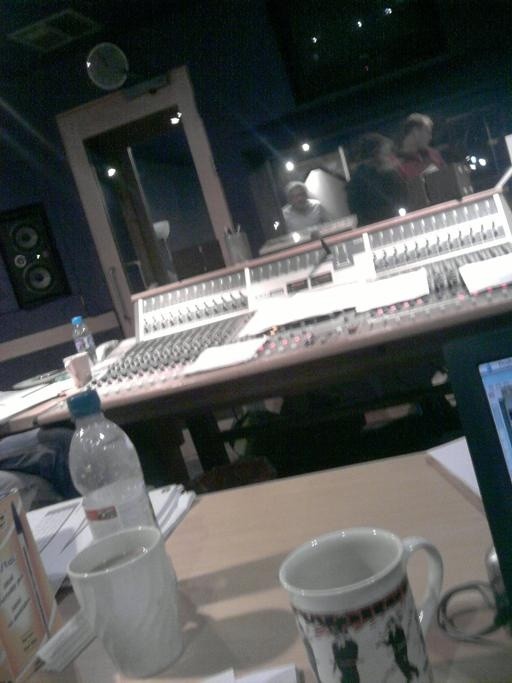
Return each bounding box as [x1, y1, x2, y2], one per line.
[373, 614, 422, 683]
[341, 128, 417, 230]
[3, 427, 107, 528]
[387, 110, 452, 213]
[328, 626, 362, 683]
[277, 178, 333, 234]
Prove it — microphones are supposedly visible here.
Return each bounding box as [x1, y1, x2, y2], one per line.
[309, 228, 335, 261]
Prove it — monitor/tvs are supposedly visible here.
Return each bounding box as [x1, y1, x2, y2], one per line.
[439, 323, 512, 612]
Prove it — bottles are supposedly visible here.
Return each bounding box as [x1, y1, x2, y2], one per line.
[65, 388, 164, 540]
[73, 314, 103, 374]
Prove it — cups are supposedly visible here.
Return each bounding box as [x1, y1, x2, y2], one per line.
[66, 525, 181, 678]
[224, 232, 252, 264]
[279, 530, 445, 682]
[64, 351, 94, 386]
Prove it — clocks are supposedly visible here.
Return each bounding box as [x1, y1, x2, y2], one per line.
[81, 40, 130, 95]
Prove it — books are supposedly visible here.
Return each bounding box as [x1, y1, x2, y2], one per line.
[17, 481, 201, 588]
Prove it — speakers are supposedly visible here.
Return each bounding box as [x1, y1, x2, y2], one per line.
[1, 200, 73, 311]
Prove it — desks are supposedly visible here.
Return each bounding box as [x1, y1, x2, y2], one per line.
[0, 292, 512, 683]
